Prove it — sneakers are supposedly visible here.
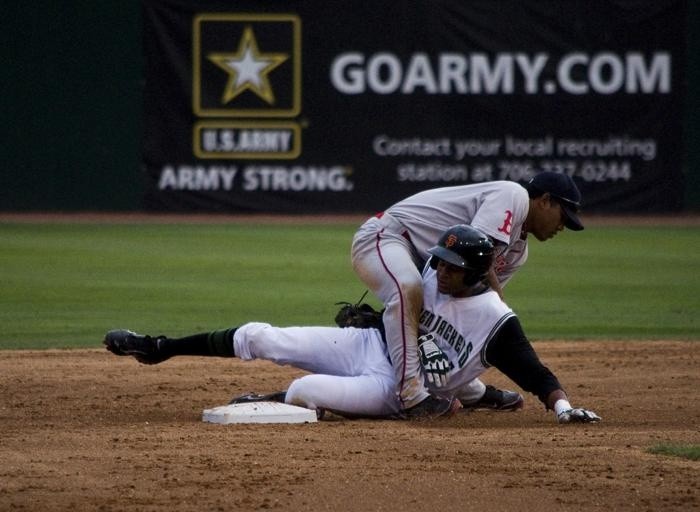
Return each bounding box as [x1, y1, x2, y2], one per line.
[103, 329, 169, 365]
[402, 394, 453, 419]
[463, 385, 523, 410]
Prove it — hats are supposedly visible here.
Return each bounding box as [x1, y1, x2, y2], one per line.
[528, 172, 585, 231]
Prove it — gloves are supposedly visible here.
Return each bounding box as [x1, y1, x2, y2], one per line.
[555, 399, 601, 423]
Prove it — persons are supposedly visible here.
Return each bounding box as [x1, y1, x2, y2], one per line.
[350, 172, 584, 419]
[104, 224, 602, 425]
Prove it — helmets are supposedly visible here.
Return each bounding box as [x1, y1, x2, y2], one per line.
[427, 224, 494, 286]
